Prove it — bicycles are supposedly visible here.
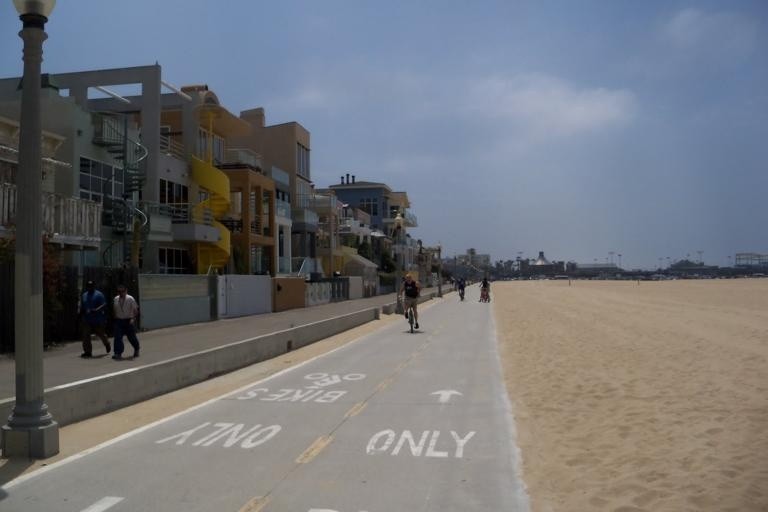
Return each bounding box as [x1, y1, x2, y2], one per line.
[478, 286, 490, 303]
[459, 288, 464, 301]
[399, 298, 418, 333]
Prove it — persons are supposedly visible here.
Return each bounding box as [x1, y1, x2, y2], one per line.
[77, 280, 111, 358]
[400, 273, 421, 329]
[111, 283, 141, 359]
[458, 277, 465, 300]
[479, 277, 490, 303]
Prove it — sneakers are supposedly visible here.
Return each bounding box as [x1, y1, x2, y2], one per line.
[415, 323, 419, 329]
[134, 347, 139, 357]
[82, 352, 92, 357]
[105, 343, 111, 353]
[112, 353, 121, 359]
[404, 312, 409, 319]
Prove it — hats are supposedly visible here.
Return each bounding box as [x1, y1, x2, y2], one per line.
[85, 279, 96, 288]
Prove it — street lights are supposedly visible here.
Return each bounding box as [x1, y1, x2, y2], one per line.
[0, 0, 57, 462]
